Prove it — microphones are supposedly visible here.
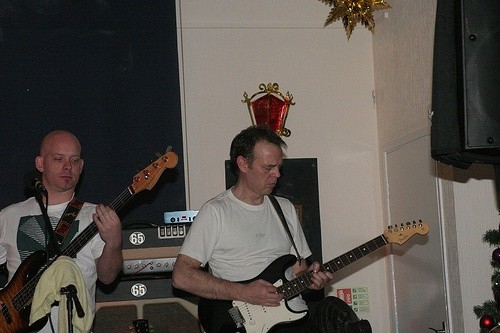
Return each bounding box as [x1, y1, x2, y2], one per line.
[30, 176, 48, 194]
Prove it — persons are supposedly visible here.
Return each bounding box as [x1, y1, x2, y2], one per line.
[172, 127, 334, 333]
[0, 130, 125, 333]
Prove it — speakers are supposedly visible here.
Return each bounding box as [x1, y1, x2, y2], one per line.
[430, 1, 500, 170]
[92, 270, 207, 332]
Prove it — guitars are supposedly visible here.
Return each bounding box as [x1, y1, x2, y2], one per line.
[197, 218, 433, 333]
[0, 144, 181, 333]
